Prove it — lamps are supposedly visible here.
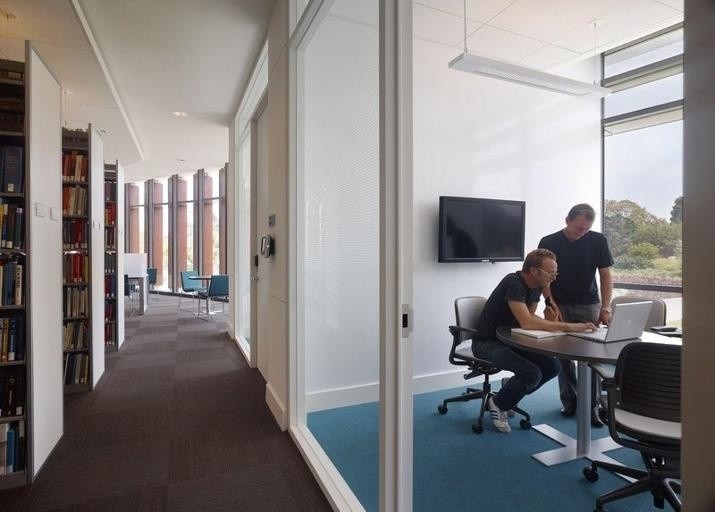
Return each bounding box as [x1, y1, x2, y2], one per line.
[448, 0, 610, 102]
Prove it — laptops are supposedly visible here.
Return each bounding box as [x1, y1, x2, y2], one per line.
[566, 300, 653, 342]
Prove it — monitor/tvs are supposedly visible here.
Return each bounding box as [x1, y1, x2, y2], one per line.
[437, 195, 526, 262]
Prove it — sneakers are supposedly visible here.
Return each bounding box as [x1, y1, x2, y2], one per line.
[488, 397, 511, 432]
[501, 378, 516, 417]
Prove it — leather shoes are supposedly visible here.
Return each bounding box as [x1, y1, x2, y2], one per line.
[592, 407, 604, 427]
[560, 406, 574, 415]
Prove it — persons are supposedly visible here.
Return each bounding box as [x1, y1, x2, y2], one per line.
[536, 203, 615, 428]
[471, 249, 599, 433]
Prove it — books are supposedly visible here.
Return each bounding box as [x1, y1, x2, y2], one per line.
[61, 150, 88, 217]
[104, 253, 116, 348]
[63, 285, 90, 385]
[511, 326, 567, 339]
[2, 61, 26, 477]
[63, 218, 90, 284]
[104, 179, 116, 251]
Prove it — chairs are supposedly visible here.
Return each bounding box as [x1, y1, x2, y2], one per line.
[147, 268, 230, 322]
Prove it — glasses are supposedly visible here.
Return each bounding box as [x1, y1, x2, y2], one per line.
[536, 267, 559, 279]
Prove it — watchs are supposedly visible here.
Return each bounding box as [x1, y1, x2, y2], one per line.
[600, 306, 612, 313]
